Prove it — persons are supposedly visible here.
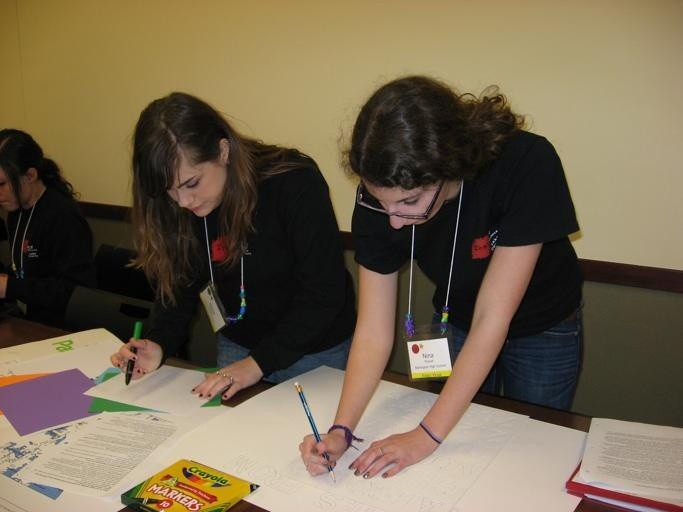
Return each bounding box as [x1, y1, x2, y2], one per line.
[0, 126, 96, 325]
[297, 74, 590, 479]
[106, 92, 358, 405]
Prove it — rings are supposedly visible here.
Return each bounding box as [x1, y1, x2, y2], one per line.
[229, 375, 233, 383]
[142, 338, 147, 348]
[215, 371, 220, 375]
[222, 373, 227, 377]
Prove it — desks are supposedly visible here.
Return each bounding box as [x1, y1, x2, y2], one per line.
[0, 314, 632, 512]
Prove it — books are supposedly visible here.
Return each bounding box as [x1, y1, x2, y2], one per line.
[0, 366, 103, 439]
[565, 413, 683, 512]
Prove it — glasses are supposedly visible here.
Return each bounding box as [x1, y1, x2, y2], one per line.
[354, 174, 446, 220]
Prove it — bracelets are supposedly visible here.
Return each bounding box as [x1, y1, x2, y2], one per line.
[418, 422, 442, 446]
[326, 424, 364, 453]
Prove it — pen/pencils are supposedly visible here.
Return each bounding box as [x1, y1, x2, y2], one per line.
[294, 382, 335, 480]
[125, 322, 142, 385]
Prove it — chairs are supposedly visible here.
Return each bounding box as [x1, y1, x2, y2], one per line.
[56, 244, 161, 344]
